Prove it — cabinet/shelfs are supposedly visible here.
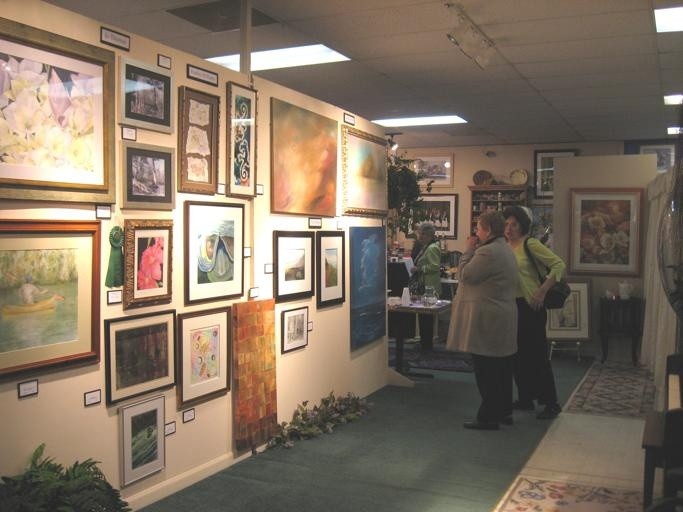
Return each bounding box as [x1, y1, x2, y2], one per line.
[466, 184, 531, 249]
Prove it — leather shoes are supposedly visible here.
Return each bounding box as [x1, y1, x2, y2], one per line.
[511, 400, 535, 409]
[537, 404, 560, 419]
[464, 420, 500, 431]
[501, 415, 512, 426]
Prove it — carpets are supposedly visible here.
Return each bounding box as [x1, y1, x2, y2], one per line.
[486, 355, 682, 512]
[387, 344, 478, 375]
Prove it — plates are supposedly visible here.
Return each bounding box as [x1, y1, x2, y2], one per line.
[509, 168, 527, 185]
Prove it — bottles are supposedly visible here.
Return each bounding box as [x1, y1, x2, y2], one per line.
[401, 287, 410, 307]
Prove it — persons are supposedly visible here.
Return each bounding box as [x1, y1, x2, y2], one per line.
[501, 206, 567, 421]
[17, 274, 50, 306]
[444, 210, 520, 431]
[409, 222, 444, 364]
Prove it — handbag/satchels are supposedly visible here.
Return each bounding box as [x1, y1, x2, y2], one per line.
[545, 282, 570, 308]
[409, 278, 425, 295]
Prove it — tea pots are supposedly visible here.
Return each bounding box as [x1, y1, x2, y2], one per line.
[618, 281, 634, 300]
[420, 285, 439, 306]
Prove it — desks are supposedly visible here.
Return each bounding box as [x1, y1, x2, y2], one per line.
[599, 295, 643, 366]
[385, 293, 453, 343]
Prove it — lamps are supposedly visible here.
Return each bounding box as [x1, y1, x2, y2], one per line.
[441, 3, 497, 74]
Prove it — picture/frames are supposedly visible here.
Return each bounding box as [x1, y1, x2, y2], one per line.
[534, 149, 581, 200]
[623, 138, 682, 174]
[568, 187, 644, 277]
[403, 193, 458, 241]
[545, 277, 593, 343]
[412, 150, 456, 189]
